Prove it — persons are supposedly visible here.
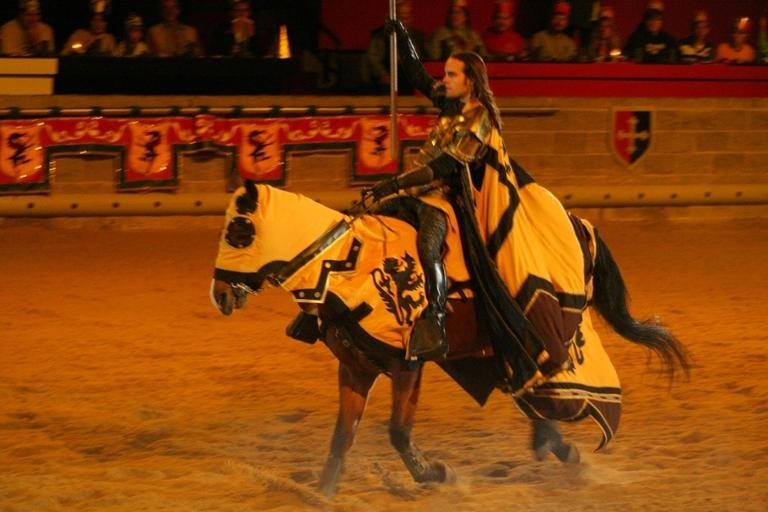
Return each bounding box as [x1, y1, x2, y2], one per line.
[582, 8, 619, 61]
[715, 14, 768, 63]
[479, 1, 529, 62]
[366, 13, 504, 360]
[528, 2, 586, 62]
[623, 1, 678, 63]
[0, 1, 270, 59]
[370, 1, 494, 95]
[676, 8, 719, 63]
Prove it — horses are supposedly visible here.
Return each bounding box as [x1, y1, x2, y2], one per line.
[210, 167, 693, 499]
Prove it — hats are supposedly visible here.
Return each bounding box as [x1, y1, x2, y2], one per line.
[123, 12, 146, 31]
[446, 1, 755, 34]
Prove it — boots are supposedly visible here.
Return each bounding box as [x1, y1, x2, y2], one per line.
[416, 259, 449, 356]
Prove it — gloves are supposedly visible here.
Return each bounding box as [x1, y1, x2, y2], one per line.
[380, 18, 410, 50]
[367, 171, 401, 203]
